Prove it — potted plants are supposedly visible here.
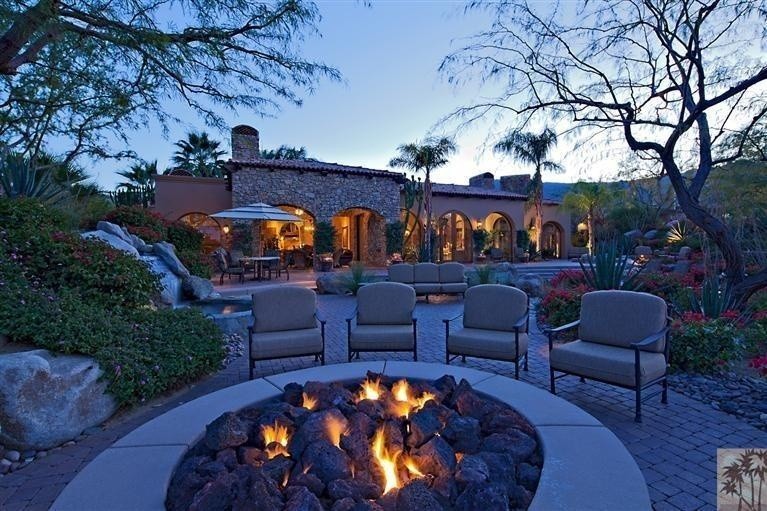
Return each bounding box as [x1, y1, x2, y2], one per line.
[471, 225, 494, 264]
[516, 230, 530, 263]
[384, 220, 405, 266]
[312, 220, 337, 273]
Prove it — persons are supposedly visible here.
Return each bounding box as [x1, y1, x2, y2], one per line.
[524, 249, 529, 263]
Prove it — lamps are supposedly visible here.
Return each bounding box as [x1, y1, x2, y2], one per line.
[222, 226, 230, 242]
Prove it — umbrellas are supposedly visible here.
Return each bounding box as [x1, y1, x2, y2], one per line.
[209, 202, 302, 278]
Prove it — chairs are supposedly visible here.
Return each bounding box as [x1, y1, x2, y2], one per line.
[387, 263, 468, 303]
[344, 281, 418, 363]
[545, 289, 672, 423]
[214, 246, 293, 285]
[246, 285, 326, 381]
[440, 284, 530, 377]
